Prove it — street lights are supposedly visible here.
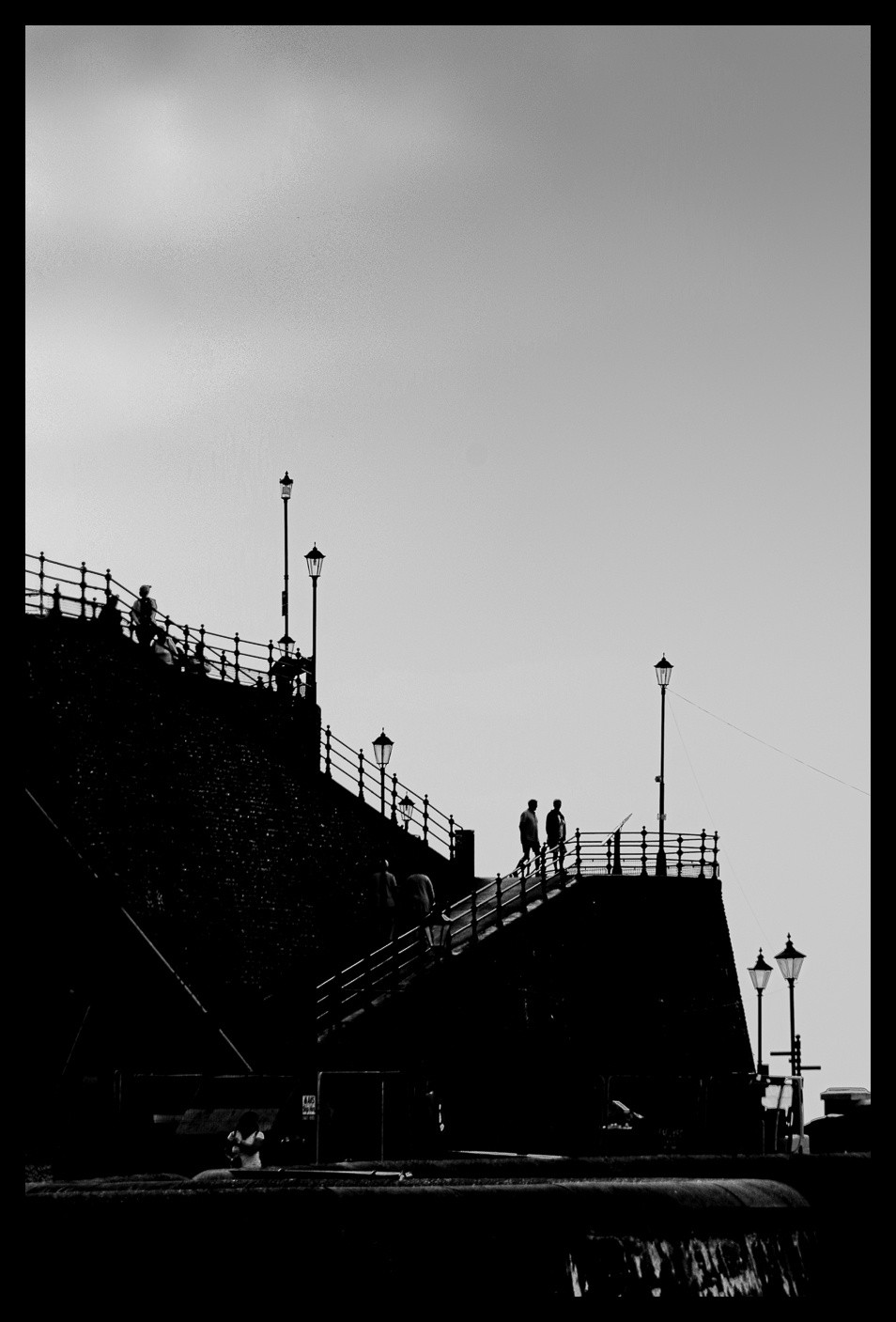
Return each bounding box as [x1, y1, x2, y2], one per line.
[304, 541, 328, 702]
[775, 933, 809, 1153]
[373, 728, 395, 813]
[398, 791, 415, 832]
[653, 652, 676, 878]
[278, 471, 296, 695]
[748, 947, 773, 1096]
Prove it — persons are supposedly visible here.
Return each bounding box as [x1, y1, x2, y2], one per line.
[373, 858, 398, 942]
[132, 585, 158, 646]
[401, 867, 436, 931]
[510, 800, 541, 879]
[226, 1111, 266, 1169]
[546, 801, 566, 874]
